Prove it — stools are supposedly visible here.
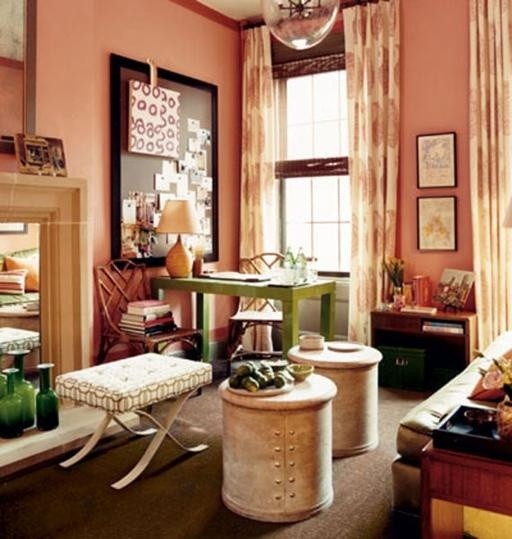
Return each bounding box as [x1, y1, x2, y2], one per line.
[286, 337, 385, 458]
[52, 350, 214, 492]
[216, 368, 339, 527]
[1, 324, 42, 372]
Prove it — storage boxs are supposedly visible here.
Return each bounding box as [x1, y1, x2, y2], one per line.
[374, 342, 427, 393]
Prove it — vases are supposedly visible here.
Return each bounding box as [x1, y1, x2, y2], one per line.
[1, 348, 35, 440]
[494, 395, 512, 439]
[390, 284, 403, 311]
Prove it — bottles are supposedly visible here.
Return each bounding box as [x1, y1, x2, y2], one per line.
[402, 281, 413, 302]
[413, 275, 432, 306]
[0, 348, 37, 439]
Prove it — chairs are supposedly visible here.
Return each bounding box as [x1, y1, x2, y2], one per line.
[90, 255, 205, 417]
[223, 250, 293, 380]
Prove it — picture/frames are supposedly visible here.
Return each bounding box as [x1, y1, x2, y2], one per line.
[415, 130, 457, 190]
[0, 0, 39, 159]
[414, 194, 458, 252]
[12, 130, 69, 179]
[104, 50, 220, 271]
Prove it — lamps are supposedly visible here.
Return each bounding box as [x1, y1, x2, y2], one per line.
[153, 196, 205, 279]
[259, 0, 342, 52]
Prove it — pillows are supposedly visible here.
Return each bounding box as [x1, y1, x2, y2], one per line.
[3, 255, 40, 292]
[1, 267, 29, 297]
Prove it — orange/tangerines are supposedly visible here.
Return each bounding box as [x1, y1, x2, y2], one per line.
[229, 362, 286, 392]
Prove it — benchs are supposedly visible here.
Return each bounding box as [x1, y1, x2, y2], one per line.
[389, 325, 511, 538]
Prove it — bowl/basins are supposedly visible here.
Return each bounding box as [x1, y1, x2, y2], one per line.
[299, 335, 326, 350]
[286, 364, 314, 381]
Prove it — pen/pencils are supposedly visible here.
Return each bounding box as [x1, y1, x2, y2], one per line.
[203, 270, 214, 274]
[245, 278, 258, 280]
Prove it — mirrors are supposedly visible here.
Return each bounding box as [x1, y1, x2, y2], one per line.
[1, 168, 93, 420]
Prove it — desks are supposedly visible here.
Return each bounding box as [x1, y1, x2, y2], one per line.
[420, 429, 512, 539]
[367, 300, 479, 378]
[147, 269, 338, 373]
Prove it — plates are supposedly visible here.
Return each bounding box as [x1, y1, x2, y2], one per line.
[226, 384, 293, 398]
[328, 342, 363, 352]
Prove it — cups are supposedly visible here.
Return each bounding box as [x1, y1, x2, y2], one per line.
[393, 295, 406, 310]
[269, 267, 318, 285]
[193, 259, 203, 277]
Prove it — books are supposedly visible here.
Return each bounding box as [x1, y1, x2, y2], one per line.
[118, 300, 178, 337]
[401, 303, 465, 334]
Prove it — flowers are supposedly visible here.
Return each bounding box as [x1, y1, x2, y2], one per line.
[377, 254, 409, 288]
[469, 343, 511, 406]
